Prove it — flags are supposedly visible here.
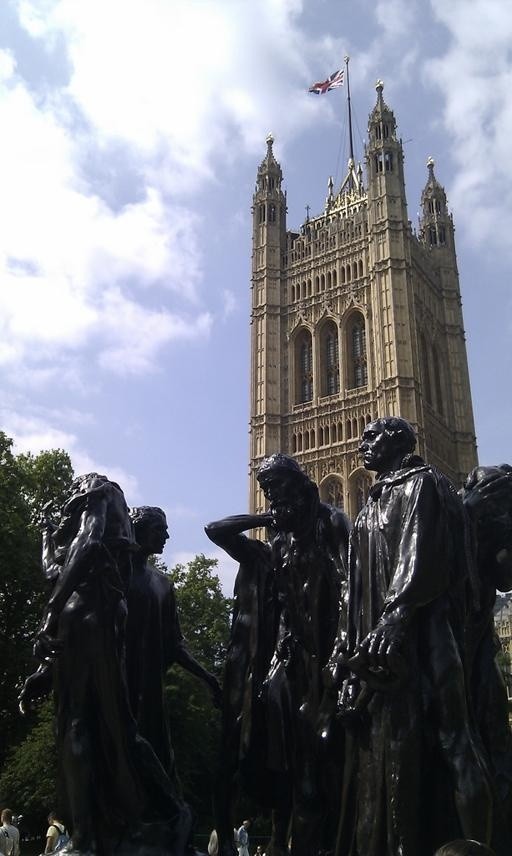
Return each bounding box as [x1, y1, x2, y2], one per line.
[309, 67, 345, 95]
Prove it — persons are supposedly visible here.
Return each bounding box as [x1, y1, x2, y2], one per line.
[17, 417, 512, 855]
[0, 808, 20, 856]
